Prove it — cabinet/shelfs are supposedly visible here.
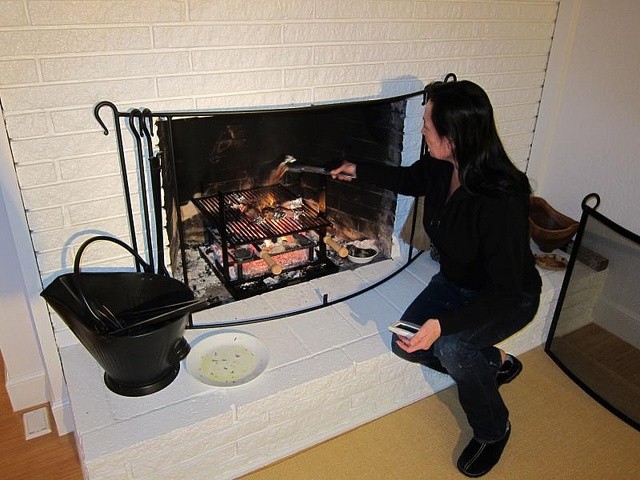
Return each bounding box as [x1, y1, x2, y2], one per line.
[191, 185, 341, 301]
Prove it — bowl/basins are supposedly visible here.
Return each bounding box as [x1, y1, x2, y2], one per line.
[343, 241, 380, 264]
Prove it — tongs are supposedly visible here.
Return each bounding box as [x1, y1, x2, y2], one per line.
[108, 297, 210, 335]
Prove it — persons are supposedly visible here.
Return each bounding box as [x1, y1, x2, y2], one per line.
[330, 76, 543, 478]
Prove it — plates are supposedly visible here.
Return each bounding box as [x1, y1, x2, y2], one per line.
[186, 332, 271, 388]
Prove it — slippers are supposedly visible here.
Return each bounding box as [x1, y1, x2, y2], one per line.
[456, 423, 511, 475]
[492, 356, 523, 386]
